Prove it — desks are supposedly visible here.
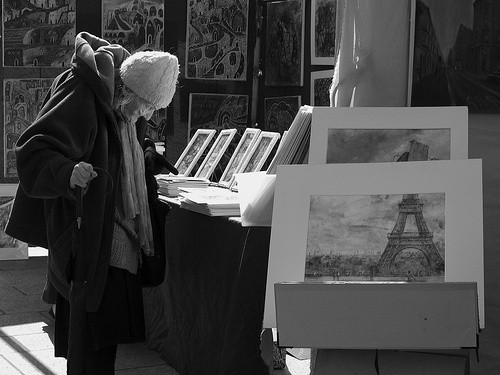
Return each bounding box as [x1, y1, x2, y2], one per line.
[143, 198, 272, 375]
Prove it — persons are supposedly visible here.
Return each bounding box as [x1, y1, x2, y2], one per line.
[3, 32, 180, 374]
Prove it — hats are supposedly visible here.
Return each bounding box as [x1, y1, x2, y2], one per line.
[119, 51, 179, 109]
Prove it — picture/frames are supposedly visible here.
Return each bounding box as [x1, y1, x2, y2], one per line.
[169, 129, 217, 177]
[194, 129, 237, 179]
[218, 128, 261, 188]
[230, 131, 281, 192]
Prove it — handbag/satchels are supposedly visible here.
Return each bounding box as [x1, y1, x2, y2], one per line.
[142, 195, 172, 287]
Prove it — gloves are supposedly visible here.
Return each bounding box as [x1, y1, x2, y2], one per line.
[144, 147, 178, 175]
